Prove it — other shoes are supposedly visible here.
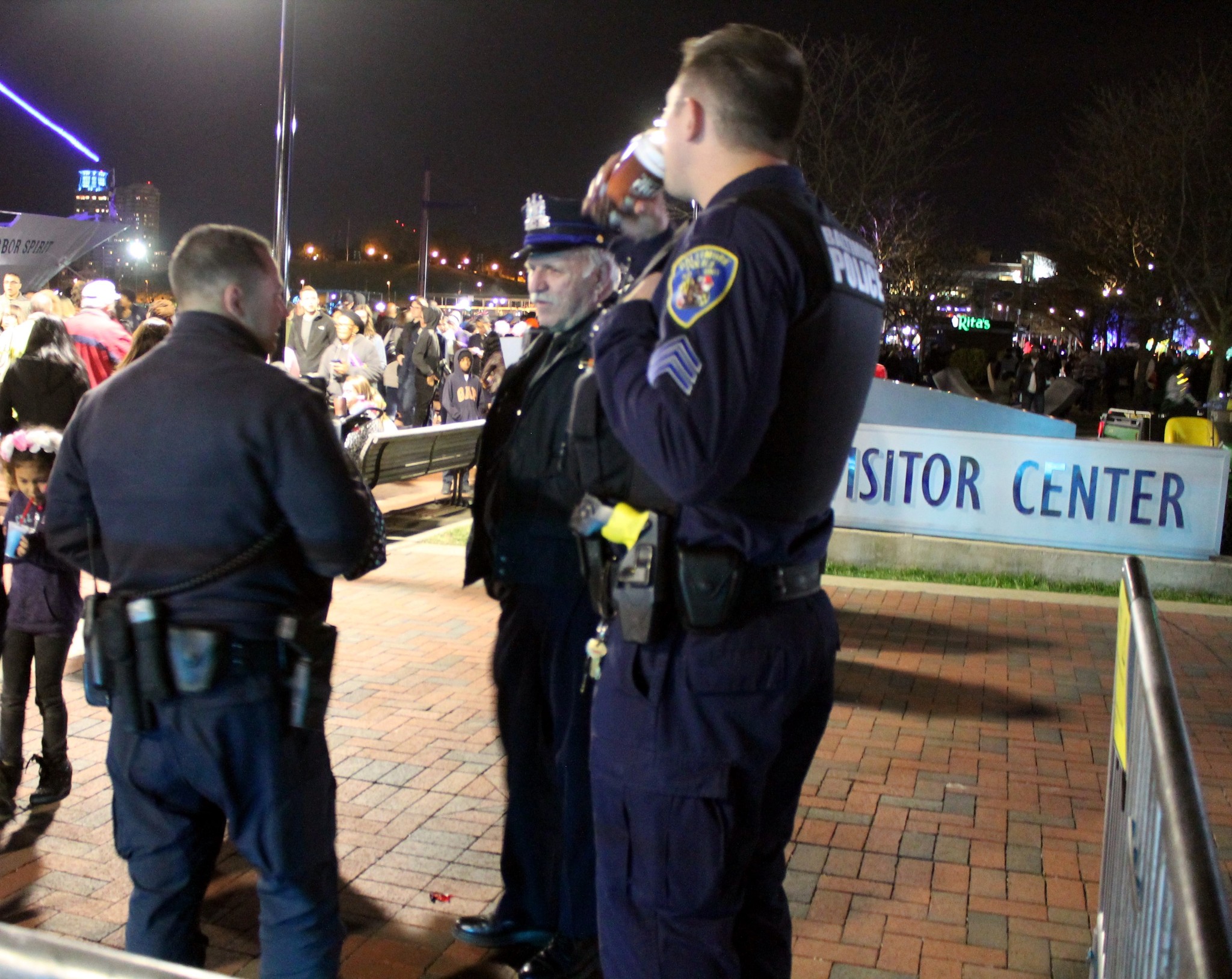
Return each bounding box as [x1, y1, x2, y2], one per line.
[441, 481, 452, 495]
[461, 480, 473, 493]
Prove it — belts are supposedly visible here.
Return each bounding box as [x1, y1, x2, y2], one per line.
[769, 560, 824, 598]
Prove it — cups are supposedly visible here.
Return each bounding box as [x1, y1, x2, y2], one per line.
[584, 130, 665, 239]
[331, 358, 343, 380]
[334, 398, 346, 417]
[5, 520, 29, 559]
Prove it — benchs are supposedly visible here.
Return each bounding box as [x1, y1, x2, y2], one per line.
[353, 420, 481, 519]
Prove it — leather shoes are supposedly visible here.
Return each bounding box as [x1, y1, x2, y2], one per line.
[457, 934, 590, 979]
[457, 901, 525, 945]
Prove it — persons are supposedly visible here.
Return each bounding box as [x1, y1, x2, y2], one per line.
[283, 282, 550, 433]
[0, 272, 180, 825]
[453, 25, 885, 979]
[43, 226, 387, 979]
[872, 278, 1232, 445]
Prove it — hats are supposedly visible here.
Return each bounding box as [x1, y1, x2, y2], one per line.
[341, 291, 355, 308]
[511, 191, 613, 261]
[340, 308, 365, 335]
[80, 278, 122, 309]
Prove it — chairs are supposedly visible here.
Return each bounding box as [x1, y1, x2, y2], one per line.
[1164, 416, 1216, 447]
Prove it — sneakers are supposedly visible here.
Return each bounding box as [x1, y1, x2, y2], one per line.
[30, 740, 72, 804]
[0, 761, 23, 825]
